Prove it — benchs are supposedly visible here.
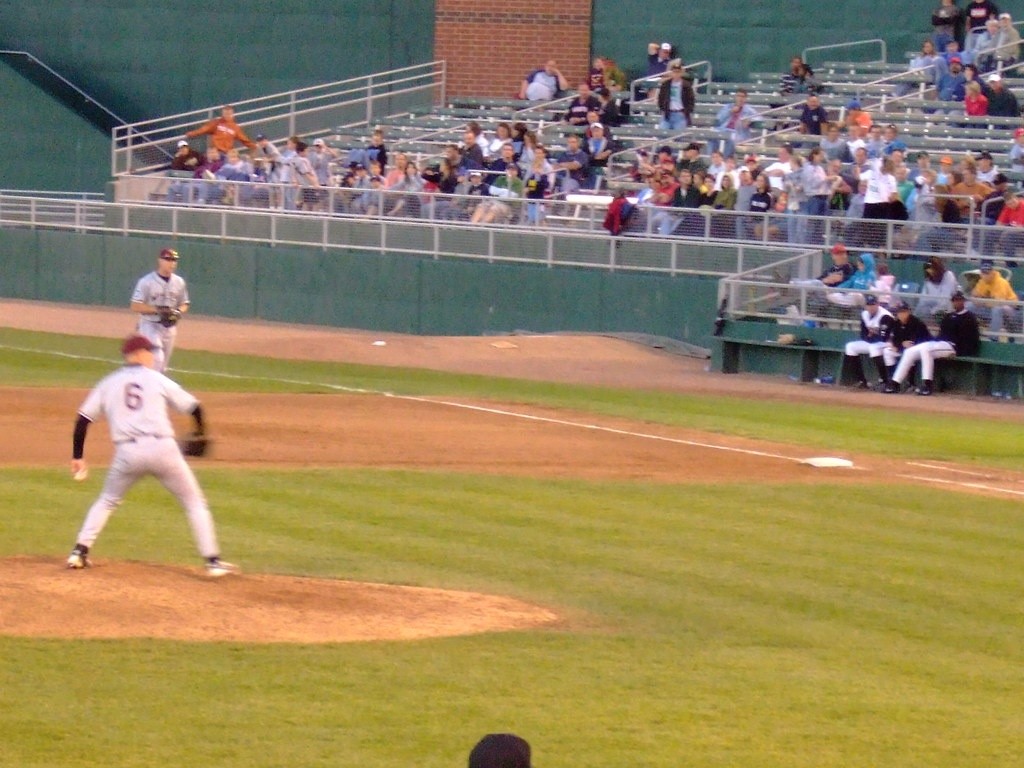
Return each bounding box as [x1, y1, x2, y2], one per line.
[713, 335, 1024, 401]
[185, 51, 1024, 263]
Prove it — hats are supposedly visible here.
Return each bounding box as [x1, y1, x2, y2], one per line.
[981, 263, 992, 271]
[469, 172, 483, 177]
[161, 248, 181, 260]
[660, 43, 672, 54]
[122, 335, 159, 355]
[866, 291, 964, 313]
[177, 140, 188, 147]
[831, 244, 847, 253]
[312, 138, 325, 146]
[256, 134, 267, 140]
[846, 101, 861, 112]
[505, 163, 516, 169]
[949, 13, 1012, 82]
[916, 151, 1008, 185]
[671, 64, 681, 69]
[470, 733, 534, 768]
[656, 146, 671, 153]
[682, 143, 699, 150]
[1016, 128, 1024, 137]
[590, 123, 604, 130]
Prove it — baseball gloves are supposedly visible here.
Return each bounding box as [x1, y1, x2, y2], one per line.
[182, 431, 210, 456]
[157, 306, 182, 328]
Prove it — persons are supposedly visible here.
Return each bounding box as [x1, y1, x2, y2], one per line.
[131, 248, 189, 373]
[470, 734, 531, 768]
[67, 334, 234, 574]
[168, 0, 1024, 394]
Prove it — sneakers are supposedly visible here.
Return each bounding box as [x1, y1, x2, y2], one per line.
[66, 549, 93, 568]
[204, 559, 243, 576]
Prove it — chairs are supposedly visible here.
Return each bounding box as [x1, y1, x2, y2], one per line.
[810, 281, 1024, 343]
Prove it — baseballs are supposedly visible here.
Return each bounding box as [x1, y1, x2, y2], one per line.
[74, 470, 87, 483]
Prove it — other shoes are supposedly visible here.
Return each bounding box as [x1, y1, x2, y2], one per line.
[874, 382, 932, 395]
